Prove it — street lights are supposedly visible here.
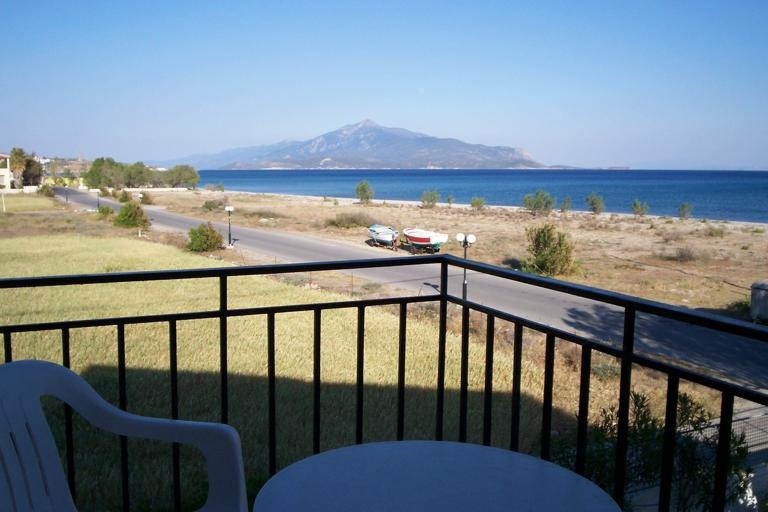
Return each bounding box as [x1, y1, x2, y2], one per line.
[456, 233, 476, 301]
[225, 206, 234, 245]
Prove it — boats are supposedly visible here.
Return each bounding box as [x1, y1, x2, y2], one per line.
[368, 224, 399, 247]
[403, 228, 448, 254]
